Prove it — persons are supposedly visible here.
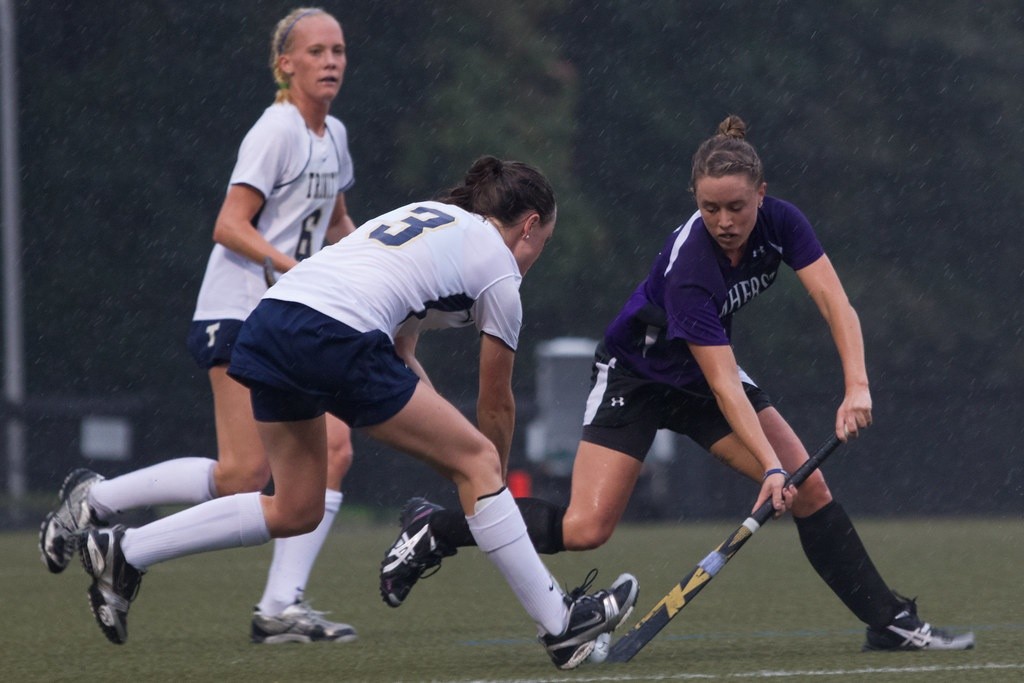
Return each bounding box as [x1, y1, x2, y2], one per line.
[379, 119, 977, 653]
[77, 157, 640, 672]
[38, 7, 359, 644]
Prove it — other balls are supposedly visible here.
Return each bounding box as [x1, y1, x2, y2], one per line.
[589, 641, 611, 665]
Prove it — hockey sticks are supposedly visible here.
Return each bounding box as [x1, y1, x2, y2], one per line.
[259, 259, 274, 290]
[605, 429, 848, 663]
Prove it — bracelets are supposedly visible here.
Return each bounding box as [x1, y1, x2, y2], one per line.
[763, 467, 791, 480]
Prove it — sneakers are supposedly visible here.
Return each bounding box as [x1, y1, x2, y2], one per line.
[866, 592, 974, 650]
[251, 600, 357, 645]
[539, 568, 639, 670]
[379, 496, 457, 607]
[77, 523, 146, 644]
[38, 468, 109, 573]
